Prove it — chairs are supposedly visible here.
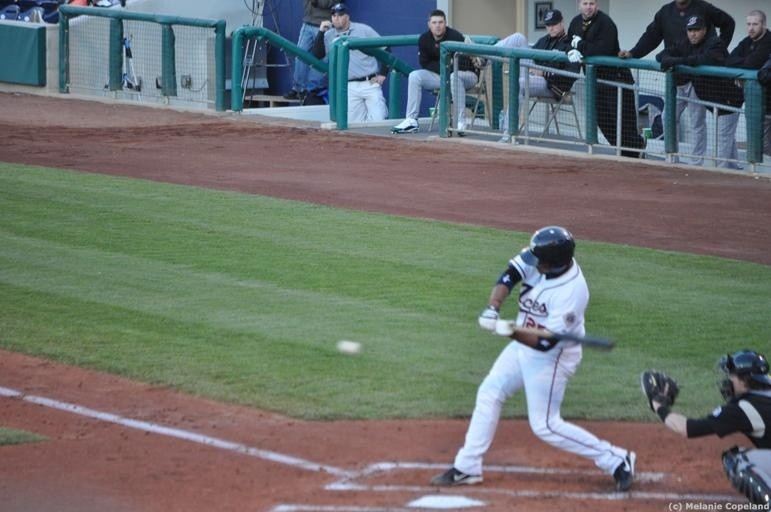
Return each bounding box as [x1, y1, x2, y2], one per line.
[429, 35, 500, 134]
[515, 64, 584, 145]
[1, 1, 62, 24]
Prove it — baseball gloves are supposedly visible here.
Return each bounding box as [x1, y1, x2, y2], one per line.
[641, 369, 680, 409]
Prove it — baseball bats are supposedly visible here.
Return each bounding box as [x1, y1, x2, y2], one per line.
[514, 327, 616, 351]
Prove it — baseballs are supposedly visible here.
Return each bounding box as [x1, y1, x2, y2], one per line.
[339, 341, 359, 356]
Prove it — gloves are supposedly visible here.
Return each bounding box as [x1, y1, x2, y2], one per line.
[567, 49, 583, 64]
[571, 35, 582, 49]
[479, 306, 514, 337]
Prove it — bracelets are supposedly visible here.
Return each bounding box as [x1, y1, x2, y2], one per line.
[657, 407, 670, 424]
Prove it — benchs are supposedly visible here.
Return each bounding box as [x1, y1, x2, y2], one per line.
[242, 95, 301, 108]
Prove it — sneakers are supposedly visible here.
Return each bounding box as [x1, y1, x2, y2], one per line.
[431, 468, 483, 486]
[391, 119, 420, 134]
[613, 449, 636, 491]
[283, 90, 303, 99]
[464, 36, 486, 69]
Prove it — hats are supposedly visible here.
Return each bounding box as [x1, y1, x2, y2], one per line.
[543, 10, 562, 24]
[687, 16, 705, 29]
[330, 4, 348, 14]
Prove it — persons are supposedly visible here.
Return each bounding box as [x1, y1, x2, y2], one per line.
[431, 224, 636, 491]
[389, 9, 480, 137]
[565, 0, 647, 159]
[617, 0, 735, 167]
[758, 59, 771, 87]
[640, 350, 771, 512]
[465, 9, 581, 144]
[655, 13, 744, 170]
[312, 3, 389, 124]
[283, 0, 343, 100]
[725, 10, 771, 156]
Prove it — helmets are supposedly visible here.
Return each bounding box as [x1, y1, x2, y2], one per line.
[719, 350, 771, 399]
[521, 226, 575, 273]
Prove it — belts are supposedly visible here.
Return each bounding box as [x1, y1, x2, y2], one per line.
[349, 74, 376, 81]
